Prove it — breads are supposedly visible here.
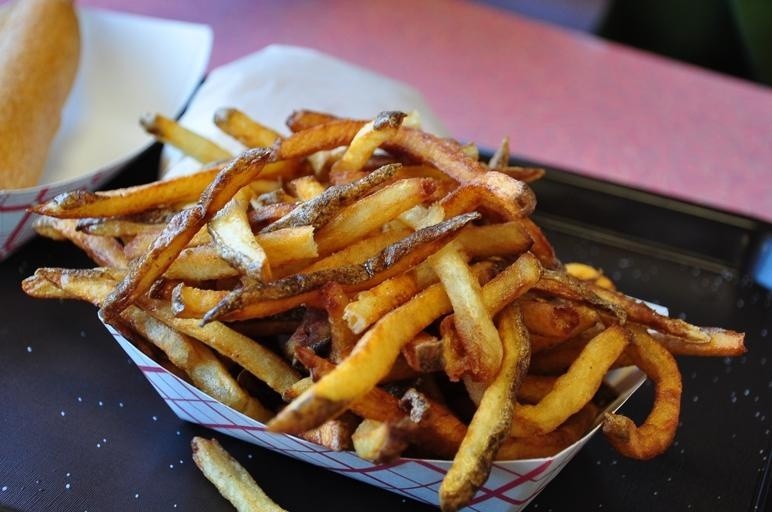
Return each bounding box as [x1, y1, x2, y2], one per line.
[1, 0, 81, 191]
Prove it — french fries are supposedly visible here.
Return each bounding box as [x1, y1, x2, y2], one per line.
[19, 108, 747, 510]
